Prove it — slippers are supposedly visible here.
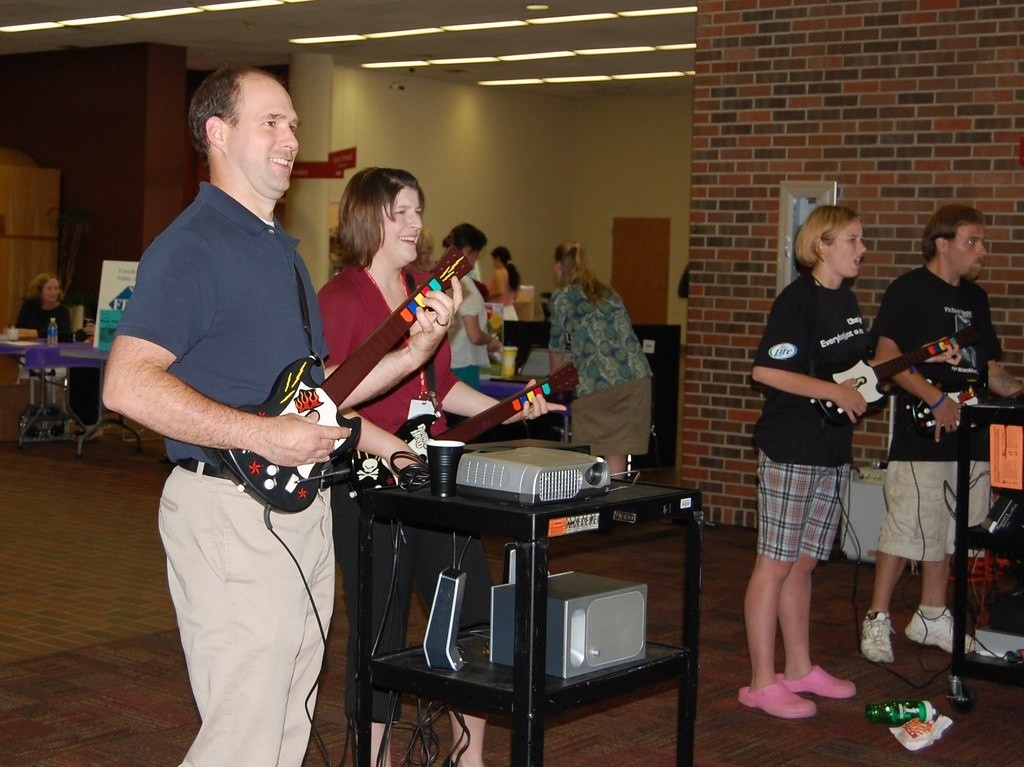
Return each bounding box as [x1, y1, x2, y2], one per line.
[775, 664, 856, 699]
[737, 682, 817, 719]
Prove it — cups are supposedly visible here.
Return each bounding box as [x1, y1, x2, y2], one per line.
[948, 678, 962, 697]
[426, 440, 465, 497]
[7, 328, 19, 341]
[502, 346, 518, 378]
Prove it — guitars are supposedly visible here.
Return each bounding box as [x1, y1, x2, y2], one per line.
[216, 243, 475, 515]
[808, 323, 981, 429]
[346, 361, 581, 527]
[907, 372, 1024, 439]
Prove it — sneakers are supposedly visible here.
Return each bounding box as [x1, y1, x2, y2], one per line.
[906, 608, 975, 653]
[860, 609, 897, 664]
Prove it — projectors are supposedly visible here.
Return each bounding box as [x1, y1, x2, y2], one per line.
[456, 446, 611, 508]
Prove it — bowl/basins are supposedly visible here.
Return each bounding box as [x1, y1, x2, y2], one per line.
[947, 685, 979, 714]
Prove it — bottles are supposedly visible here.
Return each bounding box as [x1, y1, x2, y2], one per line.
[865, 699, 936, 726]
[48, 318, 58, 346]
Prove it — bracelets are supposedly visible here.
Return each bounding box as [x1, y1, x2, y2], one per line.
[930, 392, 947, 409]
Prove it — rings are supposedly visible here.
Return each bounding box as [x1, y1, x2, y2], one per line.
[436, 317, 450, 326]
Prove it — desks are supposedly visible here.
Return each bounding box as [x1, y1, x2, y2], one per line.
[25, 341, 143, 462]
[480, 374, 571, 445]
[0, 335, 91, 448]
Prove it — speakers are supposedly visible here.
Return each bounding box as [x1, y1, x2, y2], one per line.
[491, 571, 649, 680]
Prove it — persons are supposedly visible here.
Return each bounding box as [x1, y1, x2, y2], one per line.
[14, 272, 72, 418]
[861, 203, 1024, 661]
[484, 245, 519, 321]
[102, 65, 464, 767]
[70, 322, 100, 426]
[316, 168, 566, 767]
[738, 206, 961, 718]
[445, 222, 502, 391]
[549, 243, 652, 479]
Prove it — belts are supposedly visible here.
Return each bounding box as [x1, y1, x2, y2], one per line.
[177, 456, 230, 480]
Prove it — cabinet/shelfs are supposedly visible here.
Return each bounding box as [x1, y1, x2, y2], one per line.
[346, 473, 707, 767]
[948, 398, 1024, 715]
